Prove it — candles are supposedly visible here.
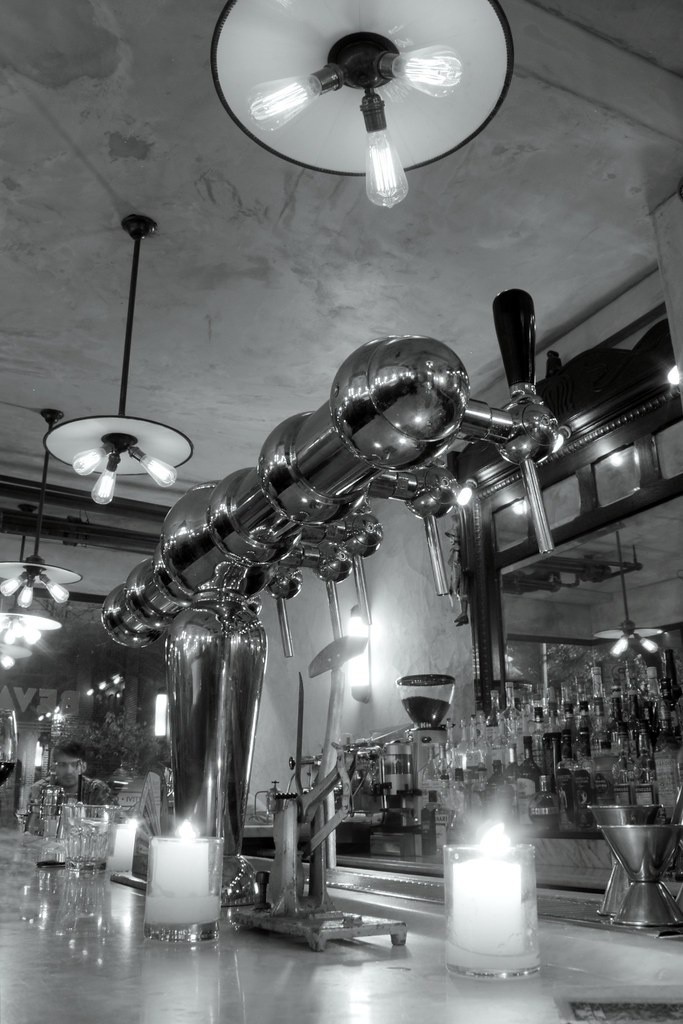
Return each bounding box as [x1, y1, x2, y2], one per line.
[115, 824, 136, 872]
[453, 859, 526, 950]
[153, 841, 208, 899]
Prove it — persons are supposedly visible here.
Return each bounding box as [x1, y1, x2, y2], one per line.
[30, 739, 116, 816]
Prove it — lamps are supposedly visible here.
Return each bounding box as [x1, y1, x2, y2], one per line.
[593, 530, 664, 658]
[0, 408, 84, 672]
[210, 0, 515, 196]
[43, 216, 194, 505]
